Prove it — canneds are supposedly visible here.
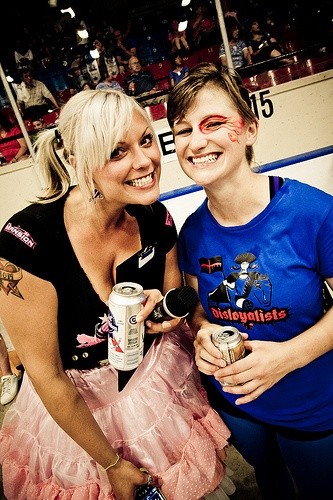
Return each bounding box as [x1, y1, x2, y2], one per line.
[211, 326, 246, 366]
[108, 282, 144, 371]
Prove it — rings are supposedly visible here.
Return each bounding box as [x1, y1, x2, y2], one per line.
[148, 476, 153, 486]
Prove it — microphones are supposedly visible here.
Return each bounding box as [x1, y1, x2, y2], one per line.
[144, 285, 200, 332]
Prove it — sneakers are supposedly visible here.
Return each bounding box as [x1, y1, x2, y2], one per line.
[0, 373, 18, 405]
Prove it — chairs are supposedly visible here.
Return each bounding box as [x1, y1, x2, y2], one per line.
[1, 26, 333, 162]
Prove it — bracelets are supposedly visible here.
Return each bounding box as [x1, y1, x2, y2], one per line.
[101, 453, 120, 472]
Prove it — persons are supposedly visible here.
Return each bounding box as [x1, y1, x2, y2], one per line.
[16, 1, 296, 131]
[167, 62, 333, 500]
[0, 89, 257, 500]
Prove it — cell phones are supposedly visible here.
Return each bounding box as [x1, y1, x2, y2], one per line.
[135, 468, 167, 500]
